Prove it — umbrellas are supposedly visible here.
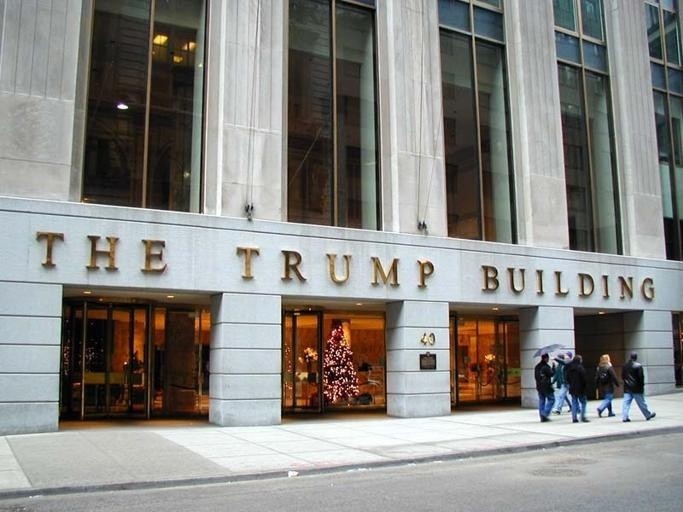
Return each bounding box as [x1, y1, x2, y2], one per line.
[532, 343, 566, 358]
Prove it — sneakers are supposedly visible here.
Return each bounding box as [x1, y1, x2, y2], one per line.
[608, 414, 615, 416]
[598, 408, 601, 418]
[646, 412, 656, 420]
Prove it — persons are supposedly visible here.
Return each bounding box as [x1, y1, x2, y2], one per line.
[549, 350, 574, 414]
[594, 354, 620, 418]
[621, 351, 656, 422]
[534, 353, 557, 422]
[551, 353, 572, 412]
[566, 355, 589, 422]
